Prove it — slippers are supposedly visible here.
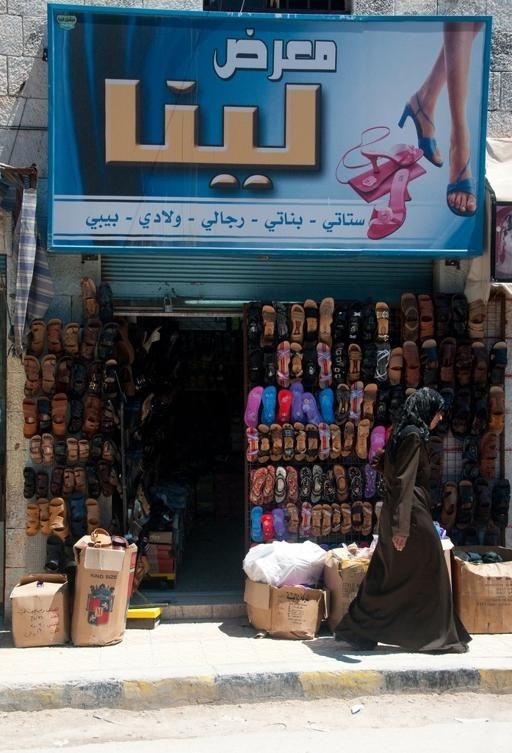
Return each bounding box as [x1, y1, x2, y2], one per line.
[22, 276, 160, 575]
[244, 291, 511, 547]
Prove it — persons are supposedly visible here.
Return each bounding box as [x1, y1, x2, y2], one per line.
[399, 20, 477, 217]
[334, 386, 471, 653]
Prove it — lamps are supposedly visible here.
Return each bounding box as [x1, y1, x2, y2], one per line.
[163, 286, 172, 316]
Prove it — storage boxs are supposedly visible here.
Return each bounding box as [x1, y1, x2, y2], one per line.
[448, 542, 512, 636]
[438, 542, 453, 596]
[63, 533, 140, 645]
[242, 574, 324, 643]
[5, 569, 69, 648]
[320, 559, 376, 635]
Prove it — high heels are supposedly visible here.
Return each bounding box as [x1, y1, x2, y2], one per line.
[397, 92, 445, 169]
[360, 143, 425, 175]
[446, 145, 477, 219]
[367, 168, 411, 240]
